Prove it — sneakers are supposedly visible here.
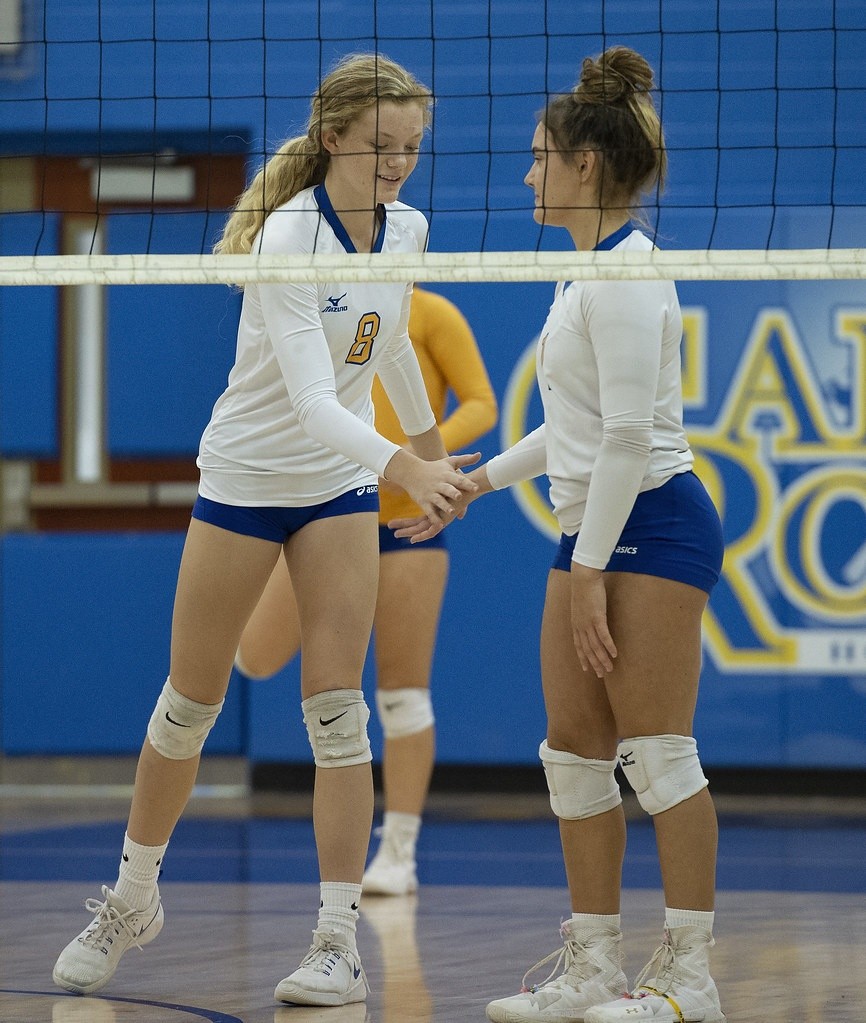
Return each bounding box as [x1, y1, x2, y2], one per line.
[273, 925, 373, 1004]
[53, 883, 164, 993]
[485, 921, 629, 1023]
[587, 916, 726, 1023]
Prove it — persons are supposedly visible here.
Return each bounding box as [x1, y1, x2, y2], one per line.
[385, 44, 728, 1022]
[54, 53, 483, 1006]
[238, 286, 500, 898]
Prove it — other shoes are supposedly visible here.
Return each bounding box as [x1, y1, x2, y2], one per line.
[360, 852, 418, 895]
